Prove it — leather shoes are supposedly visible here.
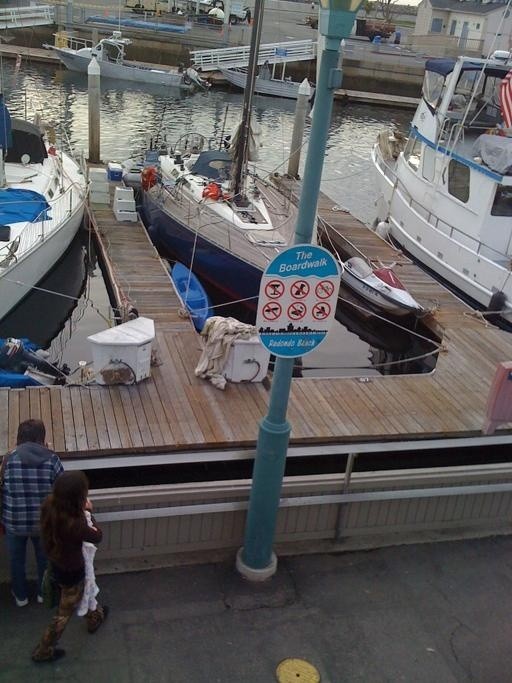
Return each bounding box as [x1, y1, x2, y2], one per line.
[32, 648, 65, 663]
[87, 605, 108, 633]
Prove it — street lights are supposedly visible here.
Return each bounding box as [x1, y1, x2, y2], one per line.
[233, 0, 364, 580]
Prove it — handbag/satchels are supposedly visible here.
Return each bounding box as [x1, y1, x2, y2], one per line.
[0, 456, 5, 536]
[41, 568, 58, 609]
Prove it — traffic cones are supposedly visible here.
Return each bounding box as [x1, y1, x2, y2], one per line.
[228, 21, 232, 31]
[103, 6, 109, 16]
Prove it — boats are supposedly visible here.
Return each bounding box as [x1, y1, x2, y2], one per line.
[216, 59, 317, 102]
[42, 0, 213, 90]
[0, 231, 86, 353]
[369, 0, 511, 327]
[0, 85, 92, 326]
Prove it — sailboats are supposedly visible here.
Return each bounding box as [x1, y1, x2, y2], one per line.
[138, 4, 324, 322]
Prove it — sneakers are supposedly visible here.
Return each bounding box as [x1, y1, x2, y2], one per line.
[11, 587, 29, 606]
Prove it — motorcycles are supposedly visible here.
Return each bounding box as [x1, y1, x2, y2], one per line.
[337, 255, 426, 317]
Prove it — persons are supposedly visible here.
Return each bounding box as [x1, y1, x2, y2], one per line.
[28, 472, 110, 662]
[1, 417, 66, 608]
